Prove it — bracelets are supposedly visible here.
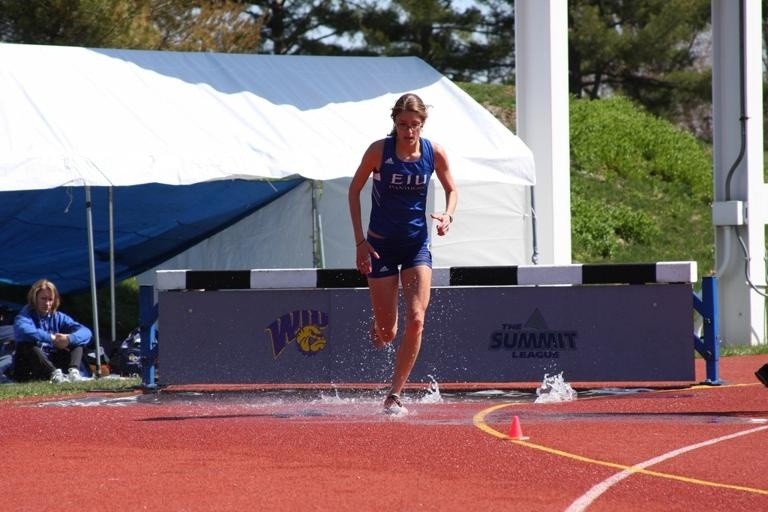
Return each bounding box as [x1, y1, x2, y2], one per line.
[443, 212, 454, 223]
[356, 238, 365, 248]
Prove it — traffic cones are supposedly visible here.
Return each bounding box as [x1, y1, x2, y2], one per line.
[504, 416, 530, 440]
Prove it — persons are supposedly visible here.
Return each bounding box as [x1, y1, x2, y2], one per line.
[12, 278, 93, 384]
[347, 92, 459, 418]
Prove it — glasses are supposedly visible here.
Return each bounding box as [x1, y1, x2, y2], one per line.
[395, 119, 425, 131]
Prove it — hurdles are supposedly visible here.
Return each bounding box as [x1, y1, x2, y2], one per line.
[138, 261, 725, 394]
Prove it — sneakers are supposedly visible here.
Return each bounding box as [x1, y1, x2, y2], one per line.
[372, 319, 385, 349]
[51, 368, 95, 384]
[382, 395, 408, 415]
